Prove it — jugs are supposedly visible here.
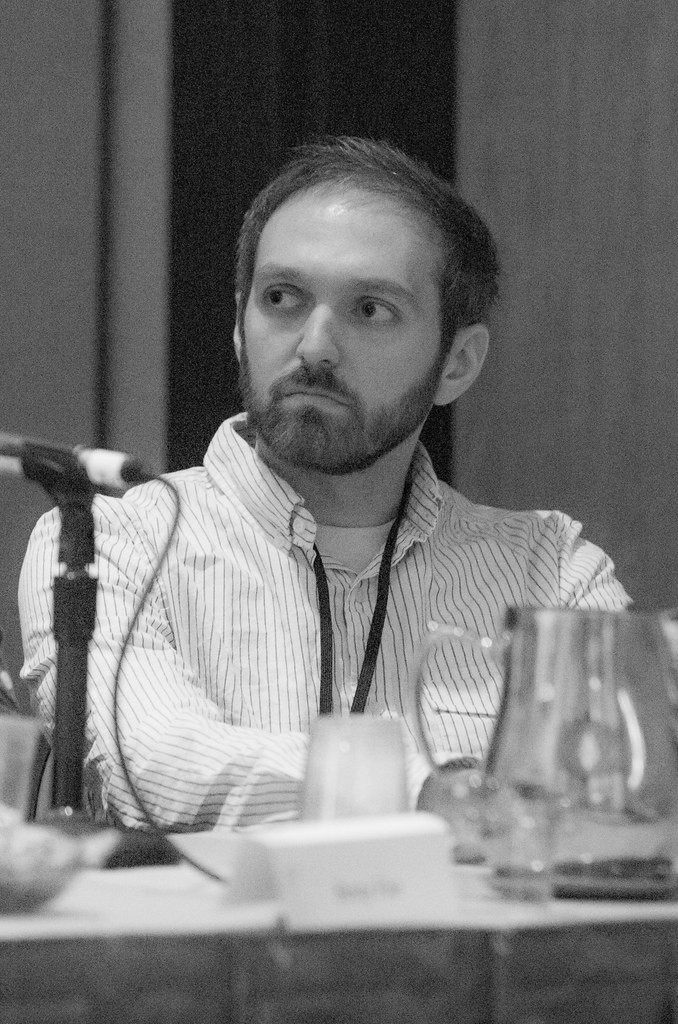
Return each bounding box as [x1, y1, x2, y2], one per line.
[412, 605, 678, 899]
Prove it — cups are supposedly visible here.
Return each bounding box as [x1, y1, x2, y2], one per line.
[299, 716, 411, 821]
[0, 717, 39, 821]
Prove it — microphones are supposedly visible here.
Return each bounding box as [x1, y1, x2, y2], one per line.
[0, 432, 153, 497]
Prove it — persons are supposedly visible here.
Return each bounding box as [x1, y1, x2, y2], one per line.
[17, 136, 649, 832]
